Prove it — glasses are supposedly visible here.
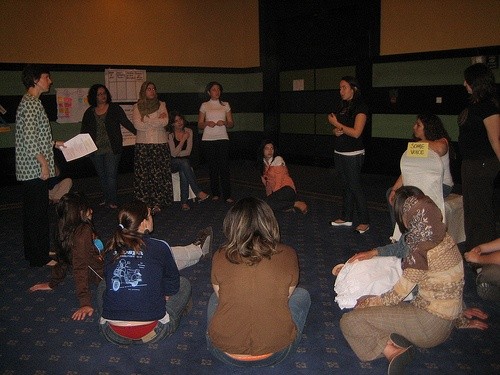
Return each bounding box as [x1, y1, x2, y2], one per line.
[96, 92, 106, 96]
[147, 87, 156, 92]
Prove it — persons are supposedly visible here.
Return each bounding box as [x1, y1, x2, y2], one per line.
[95, 205, 193, 348]
[30, 166, 211, 321]
[169, 111, 209, 212]
[15, 63, 66, 267]
[206, 198, 311, 368]
[457, 63, 500, 248]
[259, 140, 309, 215]
[132, 82, 174, 212]
[389, 111, 455, 243]
[80, 84, 137, 209]
[198, 82, 235, 202]
[329, 78, 371, 233]
[332, 186, 500, 375]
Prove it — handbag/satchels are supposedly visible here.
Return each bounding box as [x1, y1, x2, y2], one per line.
[151, 208, 160, 215]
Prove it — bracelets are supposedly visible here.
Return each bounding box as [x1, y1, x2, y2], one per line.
[391, 190, 396, 192]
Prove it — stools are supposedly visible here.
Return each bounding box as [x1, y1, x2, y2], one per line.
[444, 194, 466, 244]
[171, 171, 195, 203]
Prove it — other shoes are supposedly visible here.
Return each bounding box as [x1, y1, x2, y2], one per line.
[46, 259, 58, 266]
[389, 333, 412, 350]
[225, 195, 235, 205]
[331, 221, 352, 227]
[376, 238, 400, 247]
[292, 207, 303, 218]
[477, 282, 500, 301]
[384, 345, 415, 375]
[109, 202, 118, 209]
[301, 200, 312, 215]
[332, 263, 344, 276]
[197, 193, 210, 202]
[180, 203, 192, 212]
[202, 226, 213, 261]
[192, 228, 205, 247]
[50, 251, 57, 256]
[465, 264, 482, 282]
[211, 194, 220, 201]
[96, 202, 109, 208]
[355, 225, 370, 233]
